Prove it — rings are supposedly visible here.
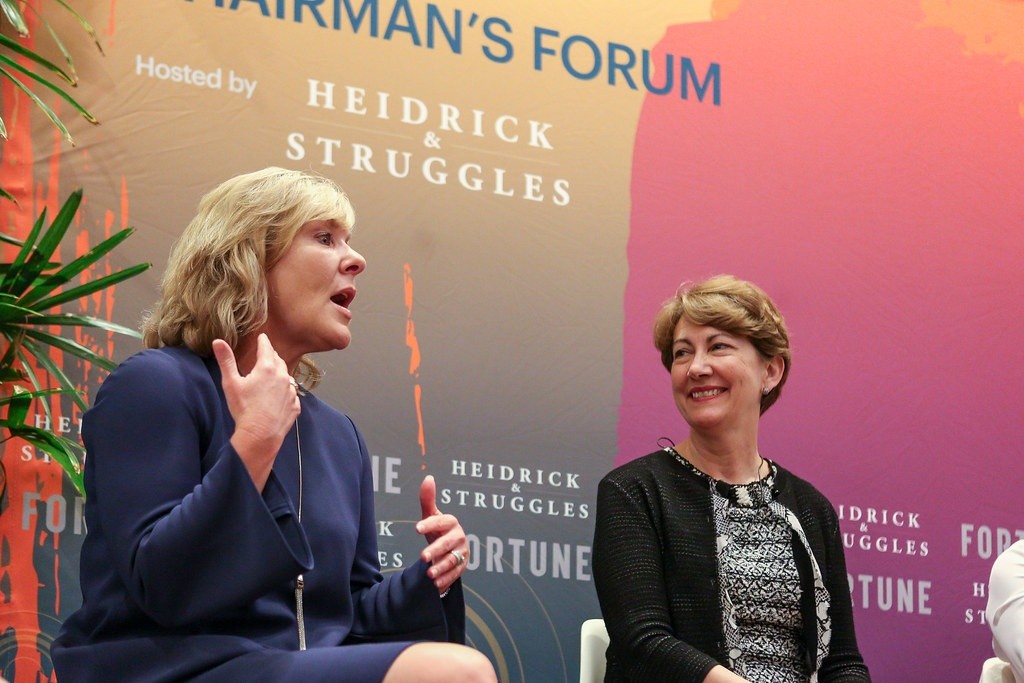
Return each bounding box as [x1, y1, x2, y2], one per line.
[289, 379, 300, 391]
[451, 550, 466, 565]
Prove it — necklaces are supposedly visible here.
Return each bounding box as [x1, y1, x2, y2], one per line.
[295, 417, 306, 651]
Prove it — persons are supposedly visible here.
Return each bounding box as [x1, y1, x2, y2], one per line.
[50, 166, 498, 683]
[986, 539, 1024, 682]
[592, 275, 873, 683]
